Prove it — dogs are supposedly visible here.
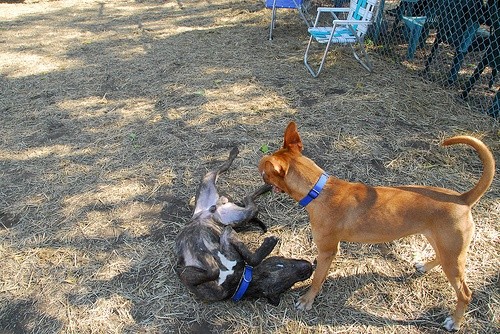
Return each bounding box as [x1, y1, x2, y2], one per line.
[174, 146, 313, 307]
[258, 122, 496, 331]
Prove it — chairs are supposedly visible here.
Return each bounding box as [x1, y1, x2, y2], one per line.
[334, 0, 386, 43]
[422, 0, 494, 84]
[460, 0, 500, 117]
[264, 0, 318, 41]
[303, 0, 379, 78]
[332, 0, 418, 55]
[381, 0, 445, 60]
